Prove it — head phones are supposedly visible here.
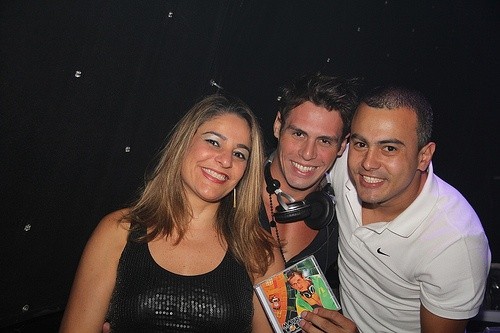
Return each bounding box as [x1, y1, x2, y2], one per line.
[300, 279, 316, 298]
[264, 153, 338, 230]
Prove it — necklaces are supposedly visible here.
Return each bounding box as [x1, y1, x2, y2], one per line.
[269, 192, 287, 263]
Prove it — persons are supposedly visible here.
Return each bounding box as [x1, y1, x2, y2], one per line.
[57, 95, 287, 333]
[286, 269, 338, 317]
[255, 70, 362, 333]
[323, 89, 491, 333]
